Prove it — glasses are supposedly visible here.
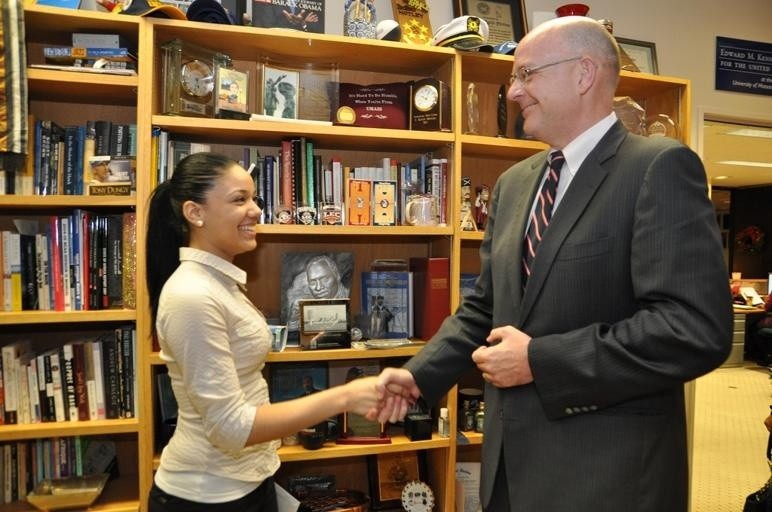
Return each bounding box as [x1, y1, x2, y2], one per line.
[505, 58, 580, 83]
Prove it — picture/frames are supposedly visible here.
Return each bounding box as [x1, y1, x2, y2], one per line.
[613, 37, 659, 75]
[453, 0, 529, 55]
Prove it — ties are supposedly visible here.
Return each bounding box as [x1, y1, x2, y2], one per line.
[522, 151, 565, 295]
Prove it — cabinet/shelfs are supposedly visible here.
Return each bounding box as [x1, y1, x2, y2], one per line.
[0, 4, 146, 512]
[456, 52, 692, 512]
[146, 15, 457, 512]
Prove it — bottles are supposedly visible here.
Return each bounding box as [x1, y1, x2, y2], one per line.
[460, 400, 474, 429]
[475, 402, 486, 433]
[438, 407, 450, 438]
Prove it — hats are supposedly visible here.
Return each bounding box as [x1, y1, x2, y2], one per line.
[112, 0, 188, 19]
[431, 15, 493, 53]
[186, 0, 238, 25]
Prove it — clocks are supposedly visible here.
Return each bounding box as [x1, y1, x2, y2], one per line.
[181, 60, 215, 99]
[718, 280, 772, 368]
[415, 85, 439, 111]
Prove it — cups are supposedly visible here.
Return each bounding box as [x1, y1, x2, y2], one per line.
[297, 202, 319, 224]
[272, 204, 293, 224]
[265, 325, 287, 353]
[321, 201, 343, 225]
[406, 193, 437, 225]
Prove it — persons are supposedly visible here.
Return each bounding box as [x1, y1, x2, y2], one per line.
[299, 375, 321, 398]
[252, 0, 320, 32]
[370, 295, 393, 335]
[461, 176, 478, 231]
[474, 184, 490, 230]
[386, 455, 411, 489]
[145, 151, 412, 512]
[364, 14, 734, 512]
[749, 294, 772, 366]
[281, 256, 350, 332]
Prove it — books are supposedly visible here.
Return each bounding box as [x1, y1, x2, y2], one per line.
[0, 114, 138, 196]
[1, 209, 137, 311]
[2, 326, 138, 425]
[0, 435, 118, 505]
[150, 126, 449, 229]
[28, 33, 139, 76]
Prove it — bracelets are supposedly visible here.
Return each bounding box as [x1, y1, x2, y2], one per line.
[302, 27, 308, 31]
